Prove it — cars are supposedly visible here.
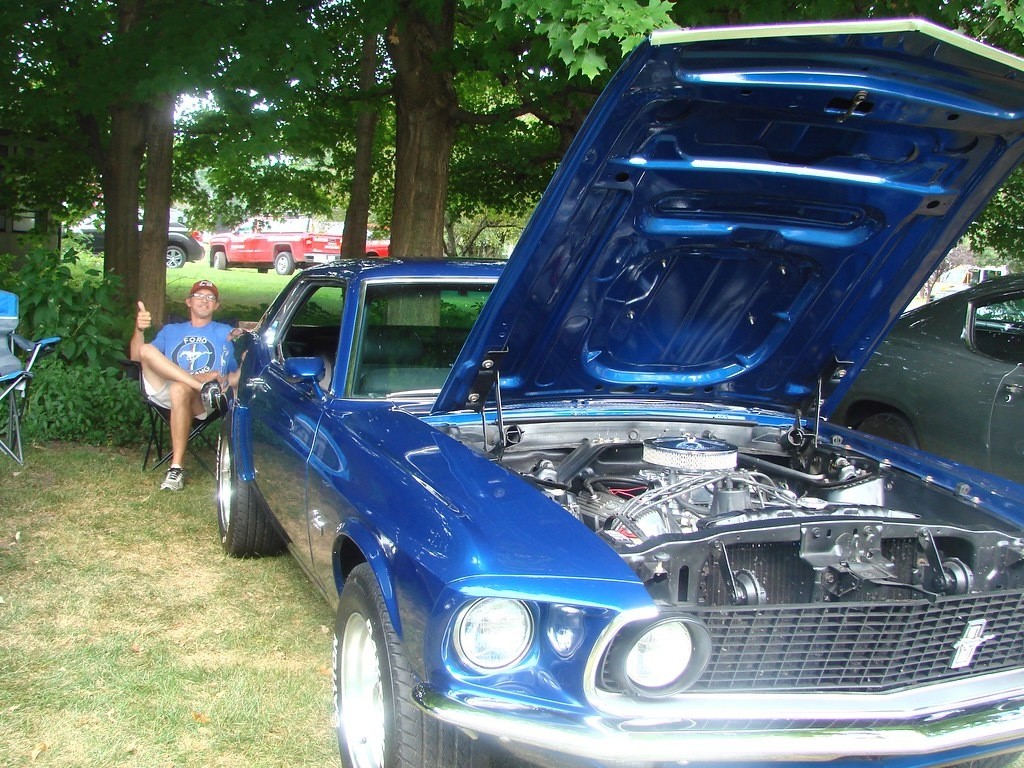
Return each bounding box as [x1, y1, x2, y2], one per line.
[828, 273, 1024, 486]
[214, 16, 1024, 768]
[209, 214, 391, 276]
[70, 208, 205, 269]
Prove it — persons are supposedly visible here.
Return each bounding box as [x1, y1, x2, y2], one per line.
[130, 280, 246, 491]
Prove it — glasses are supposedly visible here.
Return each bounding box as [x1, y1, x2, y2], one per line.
[189, 293, 217, 301]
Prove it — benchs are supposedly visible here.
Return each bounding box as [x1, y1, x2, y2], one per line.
[284, 333, 467, 368]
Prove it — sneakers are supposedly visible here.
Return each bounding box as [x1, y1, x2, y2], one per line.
[200, 382, 219, 408]
[161, 467, 184, 491]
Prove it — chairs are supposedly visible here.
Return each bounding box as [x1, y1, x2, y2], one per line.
[0, 289, 62, 466]
[116, 313, 241, 479]
[360, 327, 425, 382]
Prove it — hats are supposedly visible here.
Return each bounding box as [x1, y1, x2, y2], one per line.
[189, 280, 218, 298]
